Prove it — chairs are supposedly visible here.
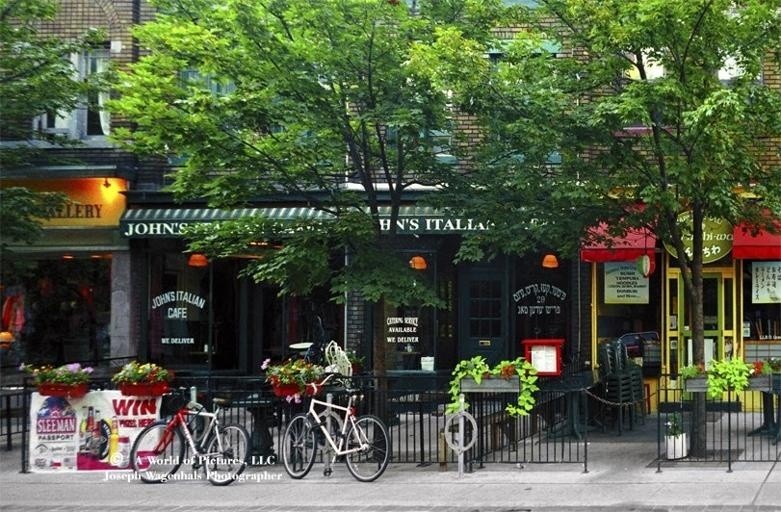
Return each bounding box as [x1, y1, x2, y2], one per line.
[600, 337, 644, 435]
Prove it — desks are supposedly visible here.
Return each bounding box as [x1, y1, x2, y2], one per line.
[223, 396, 282, 465]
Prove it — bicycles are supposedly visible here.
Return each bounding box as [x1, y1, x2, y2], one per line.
[130, 384, 252, 486]
[281, 371, 392, 483]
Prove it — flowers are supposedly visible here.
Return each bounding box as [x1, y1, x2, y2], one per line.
[666, 412, 686, 438]
[20, 360, 92, 385]
[111, 360, 176, 385]
[262, 358, 329, 394]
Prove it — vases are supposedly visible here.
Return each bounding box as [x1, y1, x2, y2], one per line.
[272, 375, 324, 398]
[120, 380, 171, 396]
[36, 378, 91, 397]
[664, 434, 688, 459]
